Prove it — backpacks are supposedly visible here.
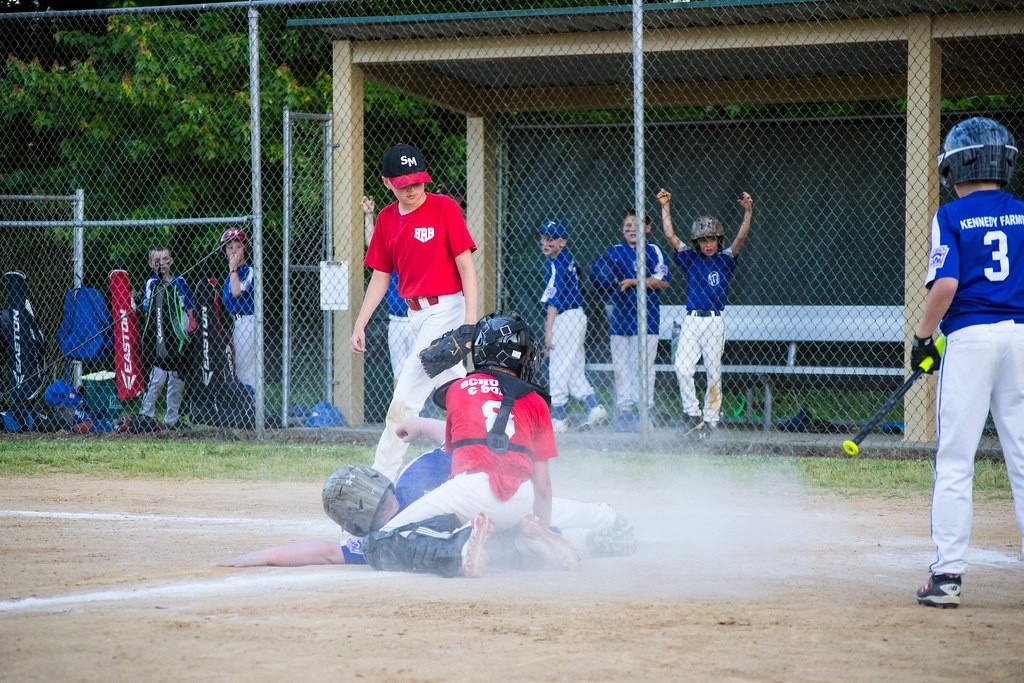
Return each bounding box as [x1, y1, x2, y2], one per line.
[143, 277, 193, 370]
[57, 286, 114, 363]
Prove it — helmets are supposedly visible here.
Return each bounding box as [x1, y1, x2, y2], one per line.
[220, 228, 252, 257]
[322, 463, 394, 538]
[691, 216, 725, 253]
[472, 309, 541, 384]
[937, 116, 1018, 192]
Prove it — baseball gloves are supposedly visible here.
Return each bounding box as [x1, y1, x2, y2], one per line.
[416, 323, 476, 381]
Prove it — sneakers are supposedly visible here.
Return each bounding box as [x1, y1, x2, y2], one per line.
[577, 403, 609, 433]
[916, 571, 962, 609]
[550, 416, 568, 433]
[615, 410, 635, 433]
[511, 513, 581, 577]
[592, 527, 638, 555]
[689, 422, 711, 440]
[679, 416, 704, 437]
[458, 512, 495, 579]
[635, 412, 654, 431]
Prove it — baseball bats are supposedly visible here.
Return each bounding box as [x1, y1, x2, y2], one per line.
[842, 334, 946, 456]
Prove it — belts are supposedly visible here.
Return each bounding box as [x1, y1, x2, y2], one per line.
[404, 290, 464, 311]
[234, 314, 243, 320]
[1014, 319, 1024, 324]
[687, 309, 721, 316]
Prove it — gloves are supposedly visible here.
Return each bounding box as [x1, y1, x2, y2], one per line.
[910, 333, 941, 374]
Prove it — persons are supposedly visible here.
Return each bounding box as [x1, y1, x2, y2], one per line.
[911, 116, 1024, 608]
[657, 187, 753, 437]
[221, 227, 256, 392]
[537, 220, 607, 432]
[362, 194, 414, 390]
[351, 142, 478, 485]
[217, 417, 637, 567]
[139, 246, 195, 423]
[362, 307, 559, 576]
[590, 209, 670, 433]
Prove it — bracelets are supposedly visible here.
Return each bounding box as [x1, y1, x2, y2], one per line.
[230, 270, 237, 274]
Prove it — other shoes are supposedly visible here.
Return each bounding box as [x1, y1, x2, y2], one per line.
[166, 420, 191, 430]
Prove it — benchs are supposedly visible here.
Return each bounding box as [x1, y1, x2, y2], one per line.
[580, 301, 905, 428]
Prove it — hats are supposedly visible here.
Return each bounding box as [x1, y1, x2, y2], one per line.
[382, 143, 432, 189]
[536, 221, 569, 240]
